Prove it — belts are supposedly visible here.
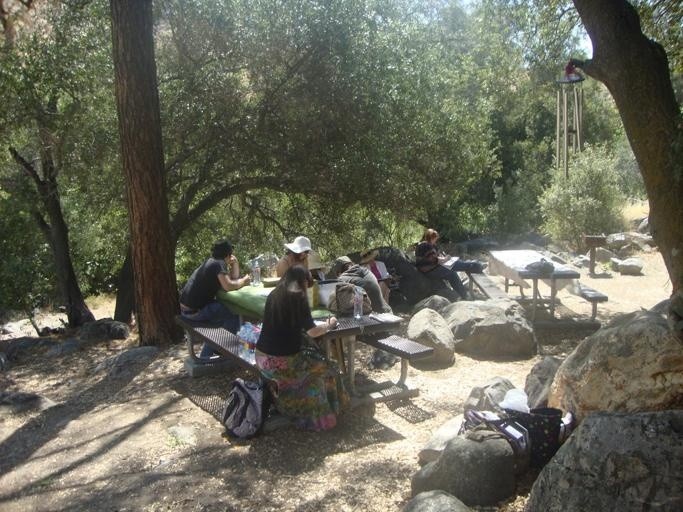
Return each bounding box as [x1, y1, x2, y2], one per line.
[180, 308, 198, 314]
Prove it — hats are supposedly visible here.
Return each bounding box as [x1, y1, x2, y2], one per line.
[334, 255, 352, 273]
[283, 235, 314, 254]
[499, 388, 531, 414]
[307, 251, 325, 270]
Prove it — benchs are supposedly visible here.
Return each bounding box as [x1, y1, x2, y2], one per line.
[566, 279, 609, 320]
[464, 270, 508, 300]
[175, 314, 290, 442]
[356, 333, 437, 399]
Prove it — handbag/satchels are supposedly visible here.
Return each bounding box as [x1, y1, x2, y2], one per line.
[328, 281, 373, 315]
[457, 408, 531, 474]
[500, 403, 563, 465]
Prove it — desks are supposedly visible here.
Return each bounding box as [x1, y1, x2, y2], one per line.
[489, 249, 580, 328]
[216, 282, 404, 414]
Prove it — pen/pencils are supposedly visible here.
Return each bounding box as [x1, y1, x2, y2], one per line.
[442, 251, 447, 256]
[329, 315, 331, 319]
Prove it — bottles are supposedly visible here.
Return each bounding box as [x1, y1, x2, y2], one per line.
[352, 288, 363, 320]
[253, 261, 260, 286]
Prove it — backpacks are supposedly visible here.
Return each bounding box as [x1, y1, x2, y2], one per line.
[221, 376, 272, 437]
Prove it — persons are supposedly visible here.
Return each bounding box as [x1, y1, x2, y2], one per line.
[272, 234, 313, 278]
[332, 256, 393, 340]
[413, 227, 487, 301]
[175, 238, 253, 363]
[358, 244, 410, 303]
[253, 264, 363, 432]
